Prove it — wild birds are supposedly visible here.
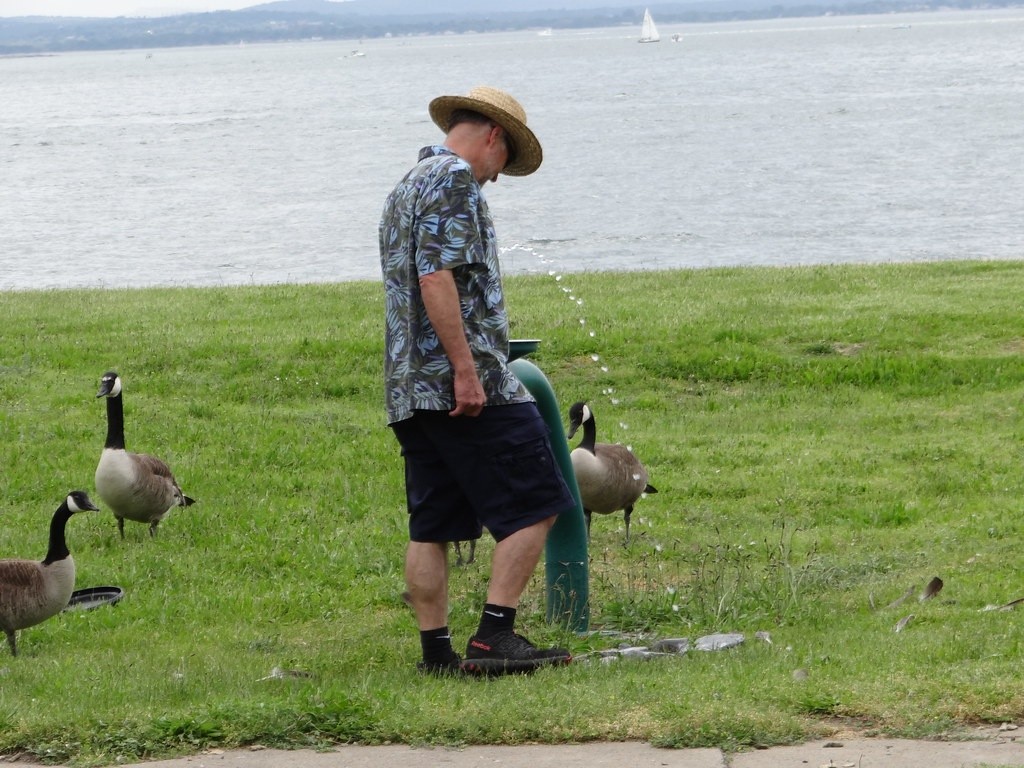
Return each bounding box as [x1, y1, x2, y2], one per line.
[453, 540, 478, 568]
[567, 402, 658, 543]
[95, 372, 196, 540]
[0, 491, 100, 661]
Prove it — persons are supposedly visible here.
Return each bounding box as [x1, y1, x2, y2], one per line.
[377, 88, 576, 678]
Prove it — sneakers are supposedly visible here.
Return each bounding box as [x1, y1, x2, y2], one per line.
[459, 629, 573, 674]
[416, 652, 464, 678]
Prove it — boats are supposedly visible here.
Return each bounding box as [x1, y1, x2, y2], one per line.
[672, 32, 682, 42]
[345, 49, 365, 58]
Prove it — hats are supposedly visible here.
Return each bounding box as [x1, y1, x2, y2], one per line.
[428, 87, 544, 178]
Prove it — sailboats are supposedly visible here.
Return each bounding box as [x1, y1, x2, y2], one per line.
[638, 7, 659, 42]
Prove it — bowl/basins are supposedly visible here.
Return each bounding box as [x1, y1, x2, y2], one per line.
[62, 586, 124, 613]
